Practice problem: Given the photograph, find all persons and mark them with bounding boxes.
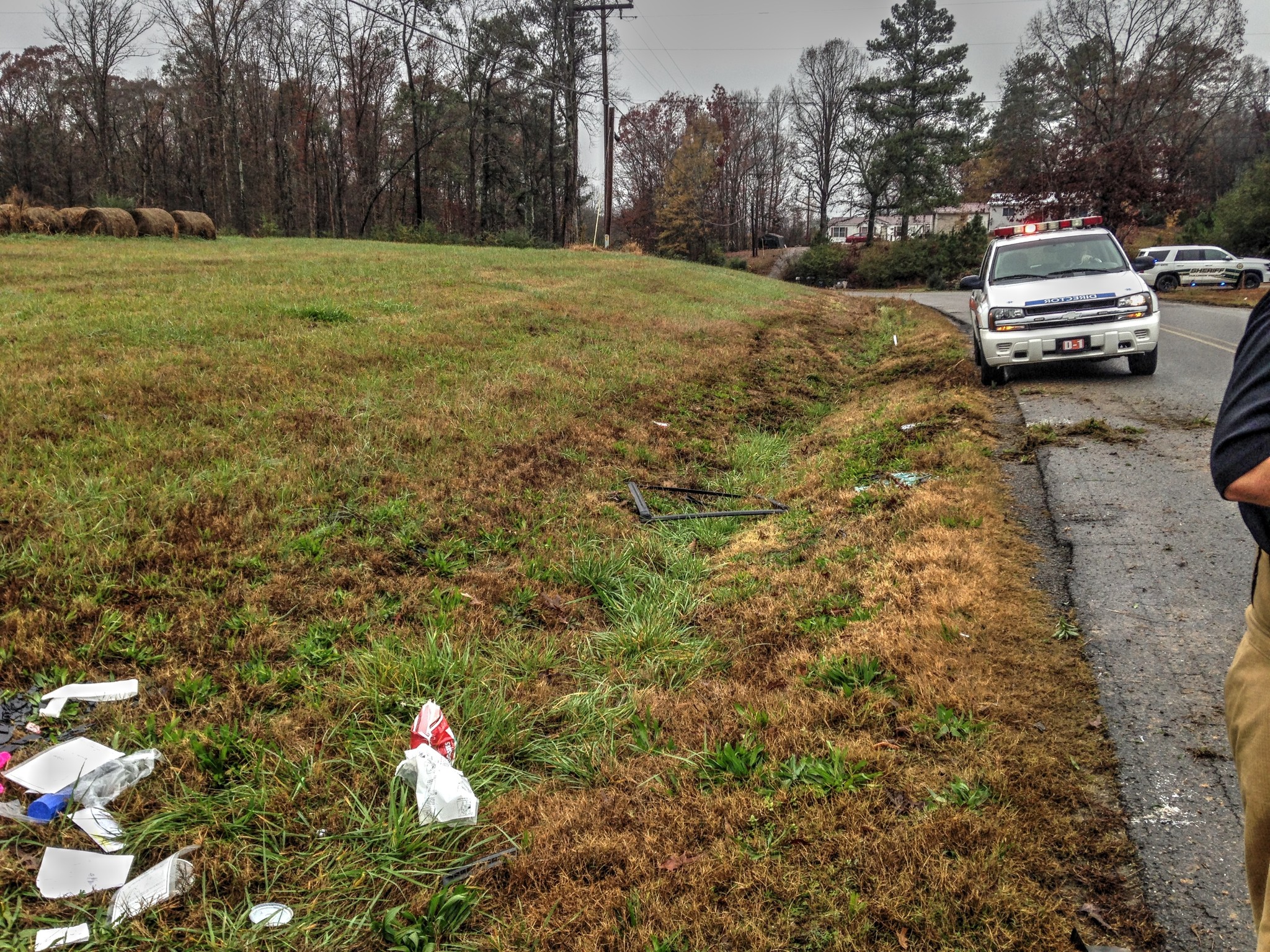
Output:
[1208,291,1270,952]
[1075,245,1102,266]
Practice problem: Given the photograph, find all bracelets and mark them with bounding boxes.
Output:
[1092,257,1095,261]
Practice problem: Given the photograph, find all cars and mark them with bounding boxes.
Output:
[958,215,1161,388]
[846,232,882,243]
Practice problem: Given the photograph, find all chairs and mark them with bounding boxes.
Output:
[998,253,1030,275]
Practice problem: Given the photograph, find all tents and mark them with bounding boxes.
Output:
[758,233,786,249]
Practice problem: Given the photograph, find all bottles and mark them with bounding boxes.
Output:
[26,793,70,824]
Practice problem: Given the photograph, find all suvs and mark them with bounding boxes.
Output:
[1137,244,1270,293]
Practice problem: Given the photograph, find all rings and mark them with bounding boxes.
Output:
[1086,257,1088,259]
